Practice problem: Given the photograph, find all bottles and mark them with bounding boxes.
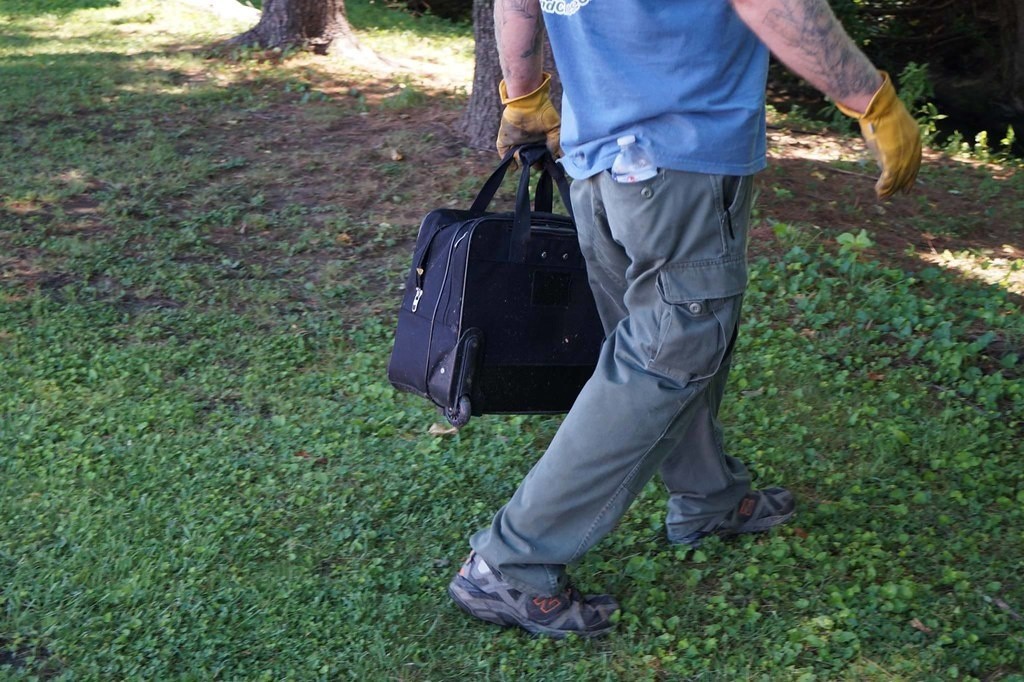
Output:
[612,134,658,183]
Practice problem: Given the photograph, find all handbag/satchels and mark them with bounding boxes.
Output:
[388,141,606,428]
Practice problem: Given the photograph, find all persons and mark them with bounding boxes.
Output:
[448,0,922,641]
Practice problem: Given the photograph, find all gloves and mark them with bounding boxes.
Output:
[833,70,921,198]
[495,69,560,161]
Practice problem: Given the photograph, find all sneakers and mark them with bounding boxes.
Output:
[448,547,620,638]
[675,486,797,543]
